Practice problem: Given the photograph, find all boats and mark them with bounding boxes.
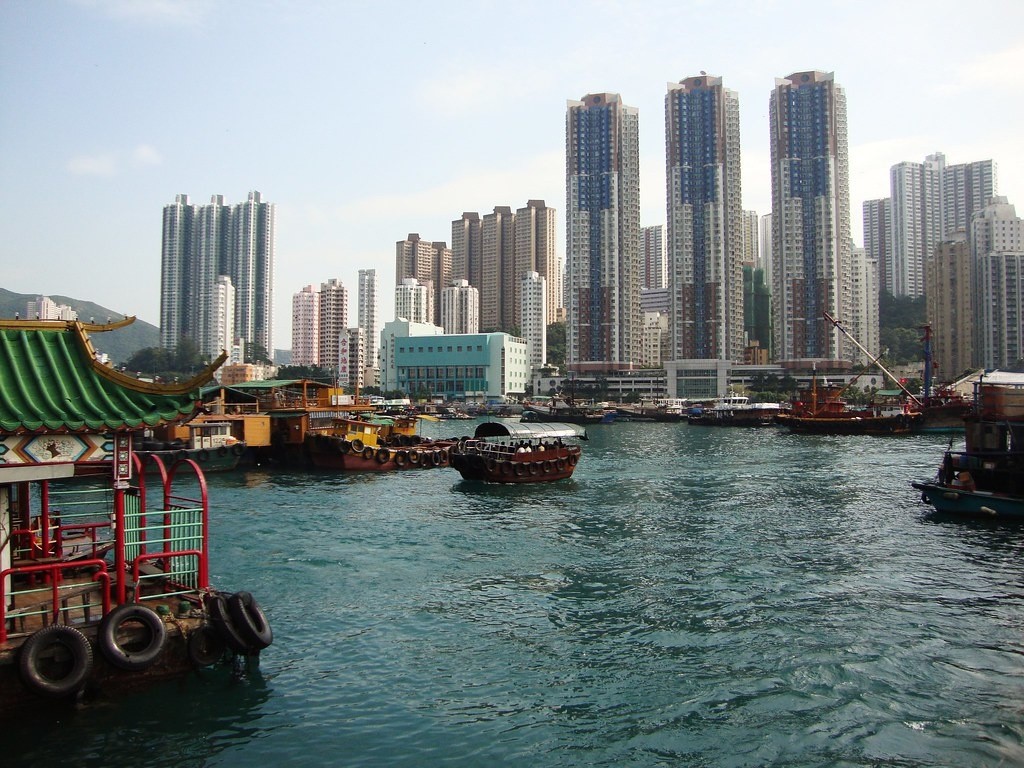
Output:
[523,398,605,423]
[911,367,1024,520]
[774,310,967,437]
[0,312,274,717]
[305,376,458,471]
[449,422,589,484]
[688,383,779,426]
[615,399,681,423]
